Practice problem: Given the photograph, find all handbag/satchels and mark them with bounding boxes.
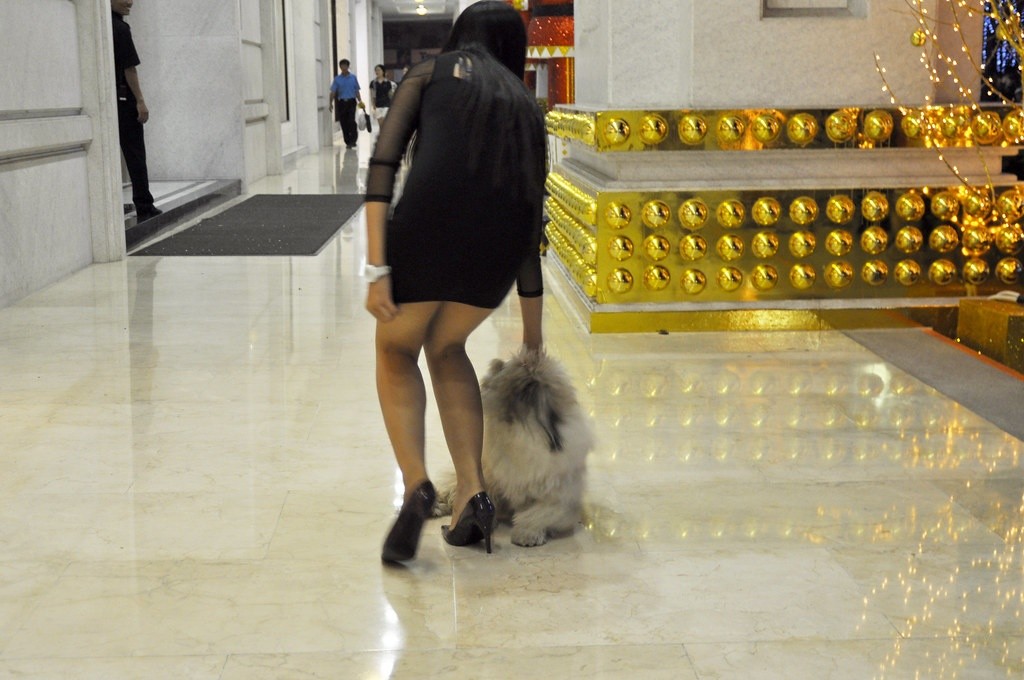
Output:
[364,109,371,132]
[359,111,367,131]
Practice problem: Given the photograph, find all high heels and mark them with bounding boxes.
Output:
[382,482,436,562]
[442,492,494,554]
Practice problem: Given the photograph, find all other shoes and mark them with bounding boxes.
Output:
[352,142,356,147]
[136,209,163,224]
[346,144,352,149]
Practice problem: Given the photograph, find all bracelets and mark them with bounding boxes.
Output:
[136,96,144,101]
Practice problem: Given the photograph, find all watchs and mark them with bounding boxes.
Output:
[364,264,392,282]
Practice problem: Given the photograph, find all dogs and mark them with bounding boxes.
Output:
[429,346,601,548]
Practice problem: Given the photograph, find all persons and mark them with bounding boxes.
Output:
[386,69,398,85]
[369,65,392,132]
[365,0,547,562]
[403,66,410,75]
[329,60,365,149]
[111,0,163,224]
